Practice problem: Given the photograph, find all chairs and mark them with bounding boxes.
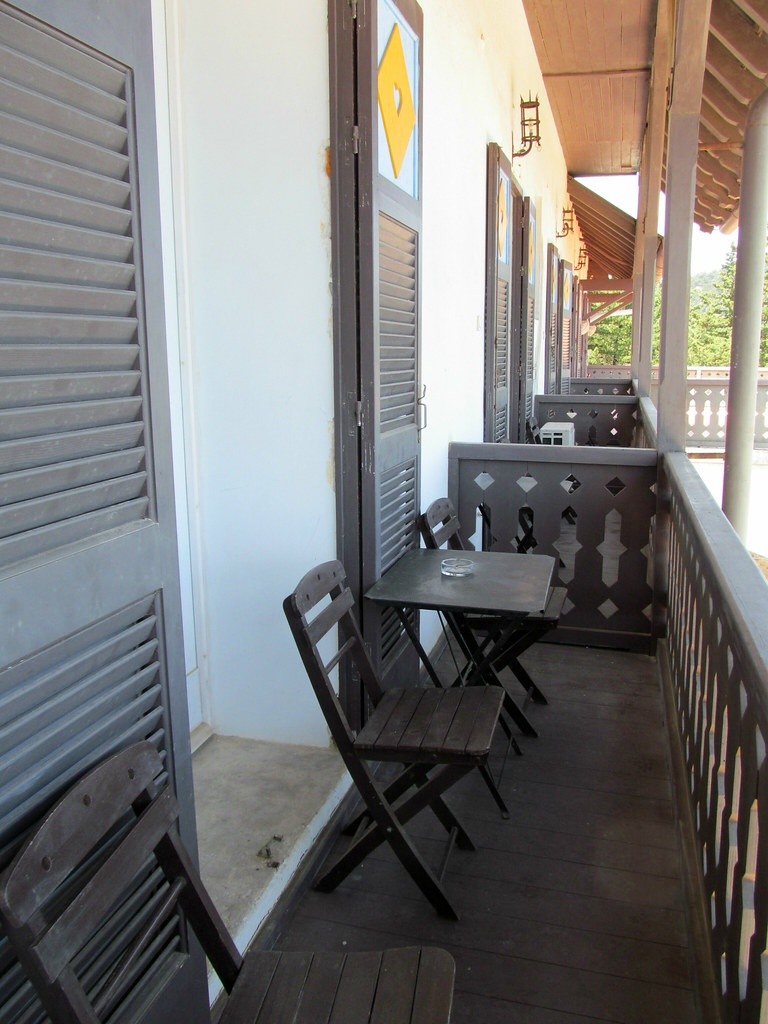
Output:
[527,416,578,526]
[416,498,569,739]
[0,740,457,1024]
[480,502,566,570]
[283,561,508,921]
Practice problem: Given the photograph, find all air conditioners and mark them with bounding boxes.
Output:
[541,420,575,447]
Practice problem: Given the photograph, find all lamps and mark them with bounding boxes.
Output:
[512,88,544,167]
[574,246,588,271]
[556,202,575,239]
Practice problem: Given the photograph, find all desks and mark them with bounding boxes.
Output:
[478,472,575,555]
[363,547,557,818]
